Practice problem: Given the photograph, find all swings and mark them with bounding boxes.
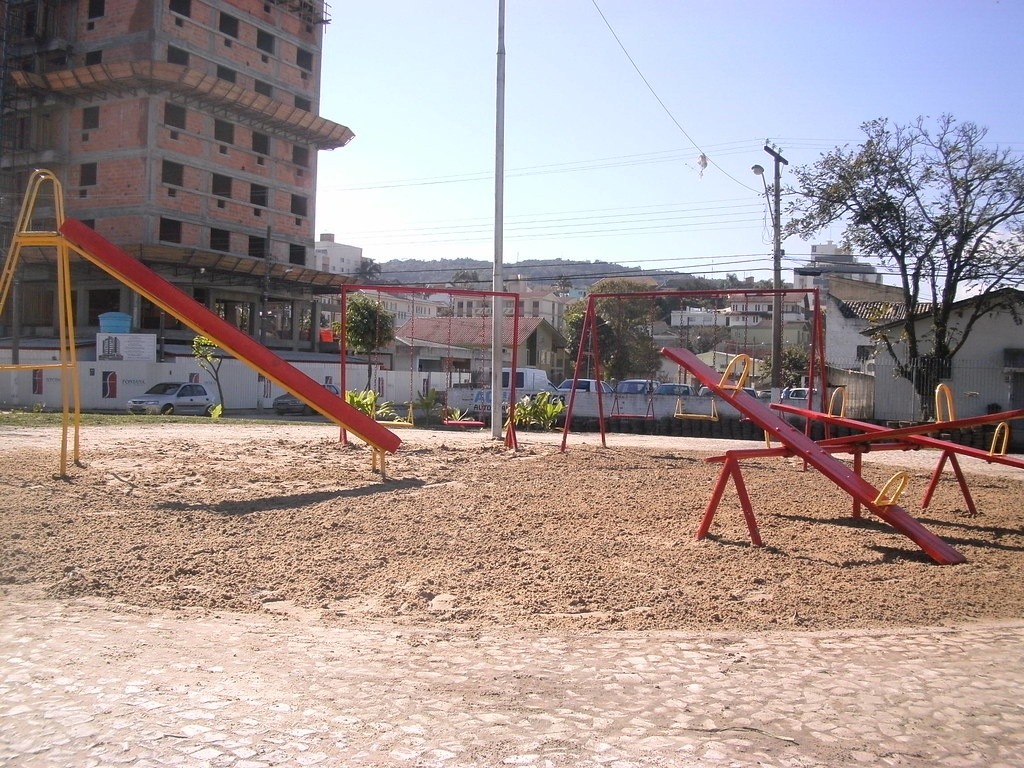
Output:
[442,293,487,427]
[673,295,719,421]
[609,293,657,420]
[739,292,786,425]
[372,288,416,429]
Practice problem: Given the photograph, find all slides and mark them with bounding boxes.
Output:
[59,215,403,456]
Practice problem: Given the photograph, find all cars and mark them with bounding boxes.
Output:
[273,383,347,417]
[698,385,758,399]
[758,390,776,398]
[557,378,614,395]
[127,381,216,417]
[612,378,662,393]
[654,383,698,397]
[789,386,817,400]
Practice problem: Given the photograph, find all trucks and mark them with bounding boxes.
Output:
[502,367,558,393]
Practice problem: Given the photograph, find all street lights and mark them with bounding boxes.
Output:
[751,136,793,407]
